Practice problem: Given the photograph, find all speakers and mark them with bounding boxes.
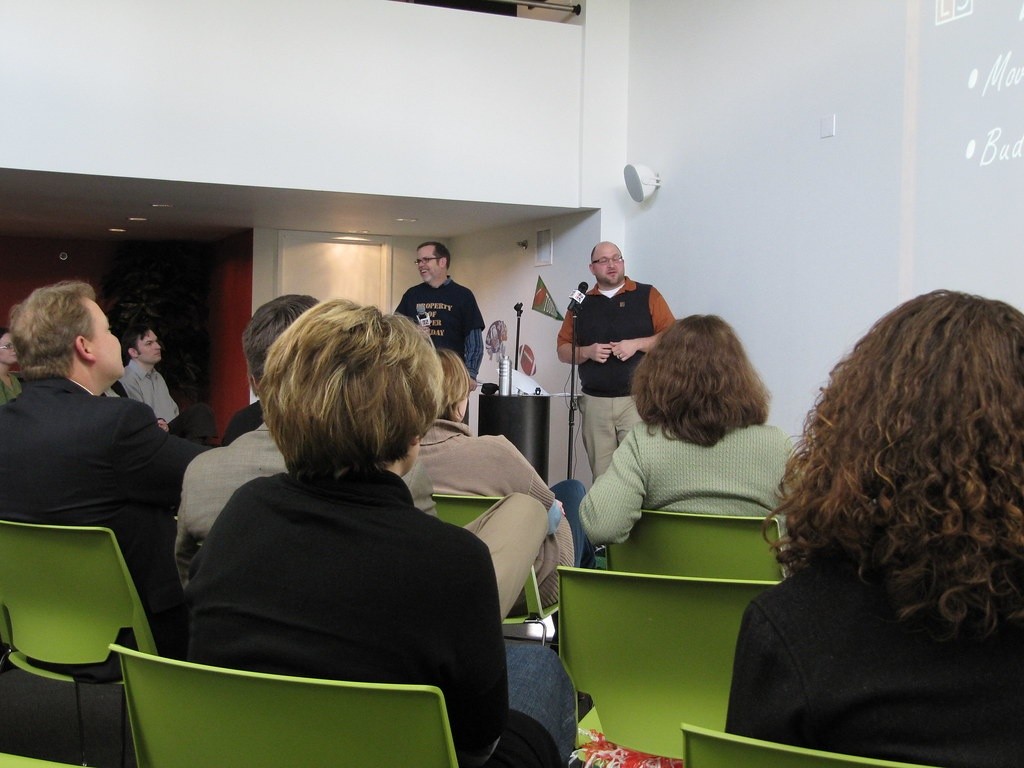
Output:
[624,164,656,202]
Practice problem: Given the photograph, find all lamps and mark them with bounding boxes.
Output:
[625,162,658,203]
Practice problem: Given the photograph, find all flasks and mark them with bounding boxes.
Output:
[498,356,512,396]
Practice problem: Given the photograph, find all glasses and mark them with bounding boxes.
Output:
[0,343,13,349]
[591,256,624,265]
[414,255,444,266]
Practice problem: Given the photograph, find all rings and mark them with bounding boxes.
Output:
[616,354,620,359]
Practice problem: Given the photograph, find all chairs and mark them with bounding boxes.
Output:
[0,513,161,768]
[680,722,943,768]
[553,565,783,763]
[430,489,558,643]
[604,498,787,586]
[106,643,462,768]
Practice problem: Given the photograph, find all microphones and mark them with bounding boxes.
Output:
[416,305,430,328]
[567,281,589,311]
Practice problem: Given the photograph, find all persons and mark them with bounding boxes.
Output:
[393,242,485,426]
[556,241,675,485]
[0,281,215,684]
[725,289,1024,768]
[417,346,587,656]
[174,294,549,624]
[579,315,796,581]
[117,323,218,446]
[0,327,23,406]
[183,297,577,768]
